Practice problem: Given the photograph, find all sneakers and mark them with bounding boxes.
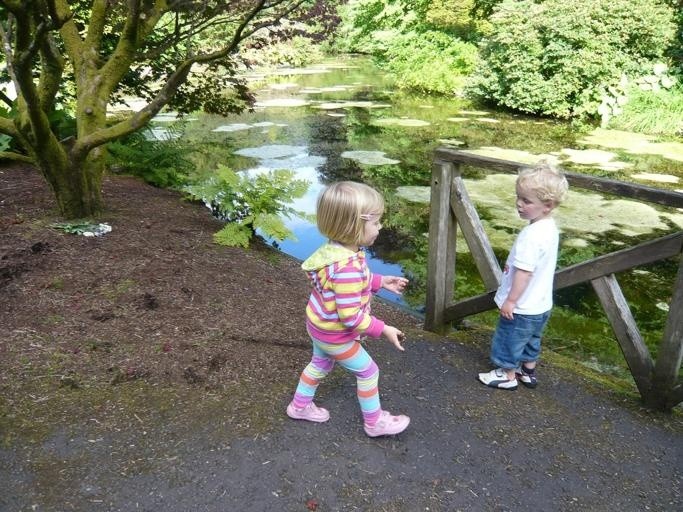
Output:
[516,365,538,389]
[476,367,519,391]
[286,400,332,423]
[362,409,411,439]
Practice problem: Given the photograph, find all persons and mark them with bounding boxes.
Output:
[477,165,569,391]
[286,181,411,438]
